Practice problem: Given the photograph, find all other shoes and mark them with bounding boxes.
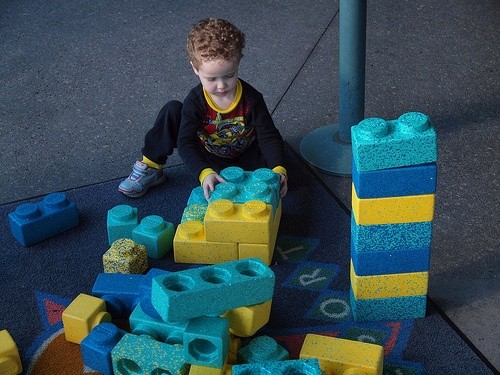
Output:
[117,160,167,197]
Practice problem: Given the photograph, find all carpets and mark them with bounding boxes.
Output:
[0,139,500,375]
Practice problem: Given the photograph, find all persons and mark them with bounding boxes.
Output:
[117,17,289,198]
